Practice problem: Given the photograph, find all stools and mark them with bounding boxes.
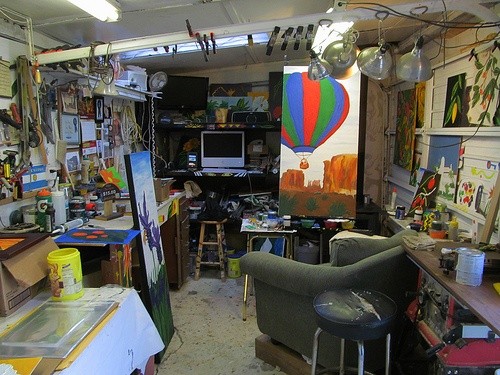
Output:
[196,219,228,281]
[312,289,397,375]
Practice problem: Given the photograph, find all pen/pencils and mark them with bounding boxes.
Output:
[88,224,102,228]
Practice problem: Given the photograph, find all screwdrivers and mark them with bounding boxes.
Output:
[3,159,10,197]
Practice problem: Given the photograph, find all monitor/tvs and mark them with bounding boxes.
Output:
[157,75,209,111]
[201,131,245,168]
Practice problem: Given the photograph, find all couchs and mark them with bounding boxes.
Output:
[241,230,418,375]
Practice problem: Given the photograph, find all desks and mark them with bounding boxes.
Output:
[0,284,166,375]
[238,207,395,265]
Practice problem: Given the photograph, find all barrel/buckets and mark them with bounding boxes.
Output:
[46,248,84,301]
[226,246,246,278]
[456,247,485,286]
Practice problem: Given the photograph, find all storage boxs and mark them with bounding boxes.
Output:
[119,71,147,91]
[154,179,176,201]
[0,237,59,318]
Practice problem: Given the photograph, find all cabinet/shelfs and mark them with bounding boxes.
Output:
[158,117,281,177]
[54,191,189,296]
[401,240,500,375]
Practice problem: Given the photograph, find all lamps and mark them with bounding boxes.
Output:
[357,43,393,80]
[68,0,123,22]
[321,40,362,80]
[307,49,334,79]
[149,71,168,91]
[397,37,434,82]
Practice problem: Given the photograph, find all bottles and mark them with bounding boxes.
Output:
[80,158,94,184]
[45,204,56,232]
[35,70,41,84]
[255,211,291,230]
[396,206,423,223]
[50,191,66,226]
[430,202,458,241]
[52,218,83,234]
[23,206,36,224]
[390,187,397,211]
[37,188,52,229]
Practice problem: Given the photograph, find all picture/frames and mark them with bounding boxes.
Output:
[94,96,105,123]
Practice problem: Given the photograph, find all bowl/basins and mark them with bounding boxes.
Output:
[342,221,354,229]
[301,220,315,229]
[324,220,337,229]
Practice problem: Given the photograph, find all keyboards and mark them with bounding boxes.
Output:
[201,168,247,173]
